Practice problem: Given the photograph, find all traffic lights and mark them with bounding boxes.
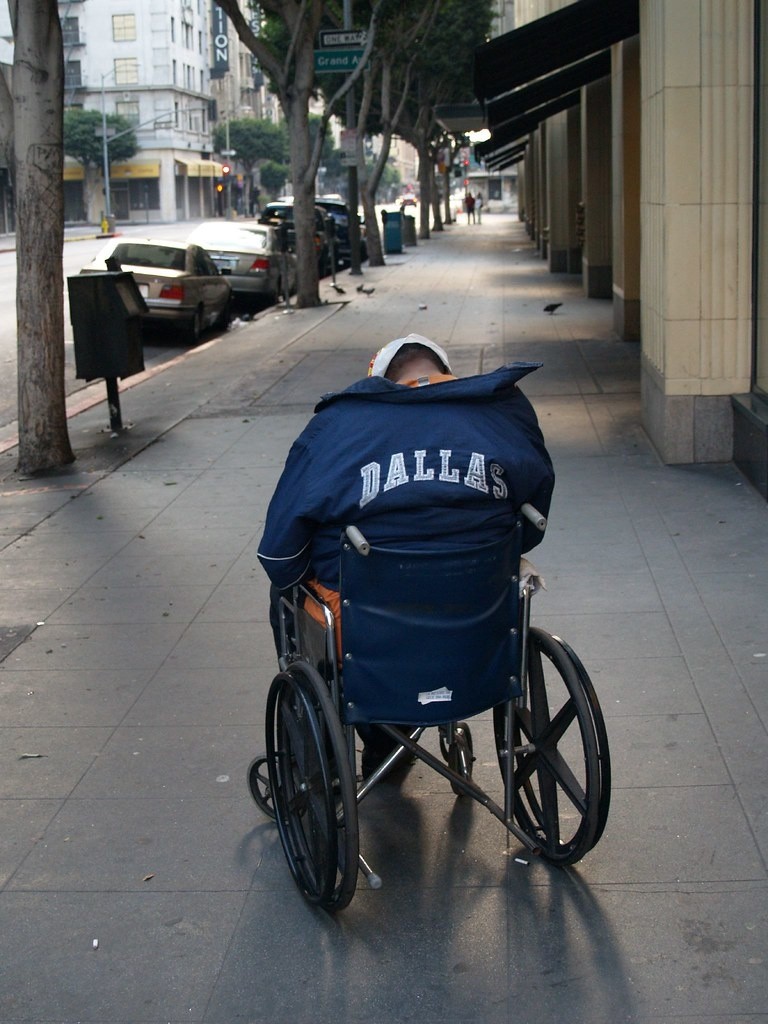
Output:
[216,181,223,195]
[222,164,232,181]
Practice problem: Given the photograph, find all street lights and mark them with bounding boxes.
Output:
[101,61,140,233]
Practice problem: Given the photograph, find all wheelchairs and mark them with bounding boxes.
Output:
[248,502,614,916]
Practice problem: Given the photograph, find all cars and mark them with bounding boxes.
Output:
[402,194,418,208]
[188,220,300,309]
[79,236,233,342]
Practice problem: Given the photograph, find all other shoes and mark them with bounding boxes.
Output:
[359,747,410,788]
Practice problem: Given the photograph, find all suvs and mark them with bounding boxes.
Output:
[315,196,368,265]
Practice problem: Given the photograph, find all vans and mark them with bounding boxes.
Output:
[256,202,344,282]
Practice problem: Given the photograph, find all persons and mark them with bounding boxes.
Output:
[465,192,476,225]
[475,193,483,224]
[256,335,557,787]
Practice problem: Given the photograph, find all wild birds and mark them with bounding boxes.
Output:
[330,283,345,294]
[543,303,562,314]
[357,284,375,297]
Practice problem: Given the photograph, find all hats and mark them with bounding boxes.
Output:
[368,332,454,383]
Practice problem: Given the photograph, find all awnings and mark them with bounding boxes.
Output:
[459,1,641,174]
